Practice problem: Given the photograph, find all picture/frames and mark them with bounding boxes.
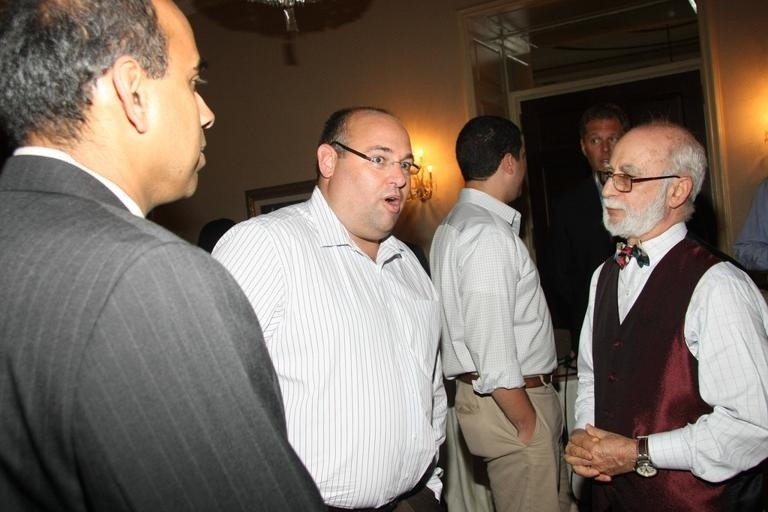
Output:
[244,178,317,219]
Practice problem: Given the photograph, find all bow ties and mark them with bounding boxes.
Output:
[613,242,649,270]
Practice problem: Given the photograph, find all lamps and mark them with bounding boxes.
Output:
[406,146,434,203]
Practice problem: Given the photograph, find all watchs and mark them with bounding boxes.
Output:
[632,435,659,479]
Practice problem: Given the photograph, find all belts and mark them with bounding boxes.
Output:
[457,373,552,388]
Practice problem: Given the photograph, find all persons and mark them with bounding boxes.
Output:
[429,115,579,512]
[540,101,717,353]
[0,0,327,512]
[563,120,768,512]
[211,106,449,512]
[730,176,768,274]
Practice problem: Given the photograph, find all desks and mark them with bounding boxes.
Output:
[434,359,597,512]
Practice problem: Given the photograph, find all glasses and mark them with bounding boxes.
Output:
[597,171,680,192]
[329,141,420,175]
